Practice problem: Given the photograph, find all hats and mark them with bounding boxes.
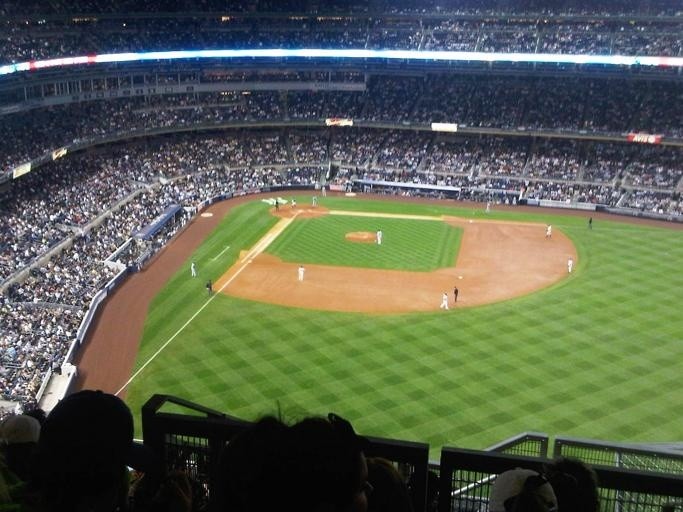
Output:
[488,467,558,511]
[0,414,41,444]
